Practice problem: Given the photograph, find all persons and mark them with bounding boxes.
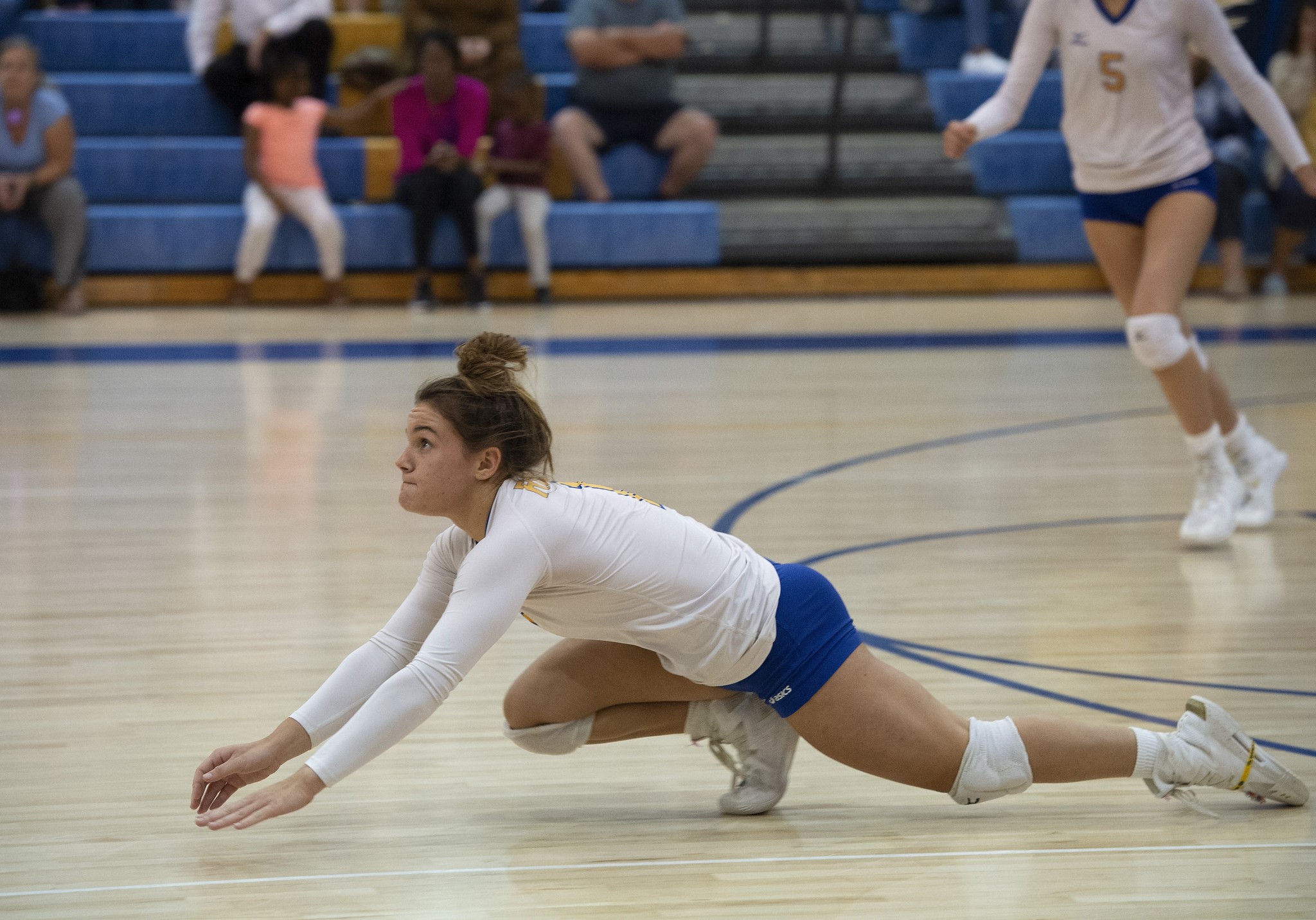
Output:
[942,0,1316,549]
[181,0,717,316]
[0,35,89,314]
[189,330,1310,832]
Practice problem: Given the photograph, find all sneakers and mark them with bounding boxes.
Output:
[1144,694,1311,819]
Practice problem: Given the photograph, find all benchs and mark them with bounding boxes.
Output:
[0,0,1298,270]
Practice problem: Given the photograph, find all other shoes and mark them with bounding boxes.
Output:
[1178,462,1244,546]
[1233,438,1289,528]
[410,280,433,308]
[528,286,550,304]
[461,274,484,313]
[212,281,255,307]
[706,691,800,815]
[325,280,351,310]
